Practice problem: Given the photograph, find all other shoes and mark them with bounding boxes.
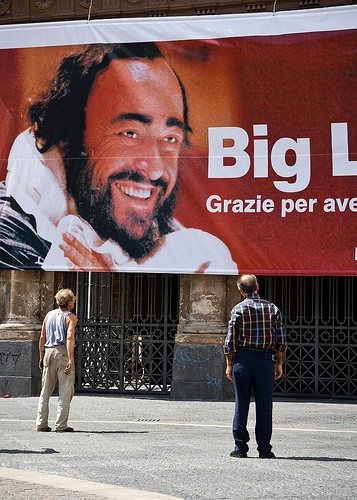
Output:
[39,427,52,432]
[259,452,275,458]
[55,427,74,432]
[229,450,247,458]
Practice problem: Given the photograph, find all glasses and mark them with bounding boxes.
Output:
[72,296,77,301]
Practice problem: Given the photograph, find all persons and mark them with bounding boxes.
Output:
[223,274,287,458]
[0,42,239,274]
[36,289,77,432]
